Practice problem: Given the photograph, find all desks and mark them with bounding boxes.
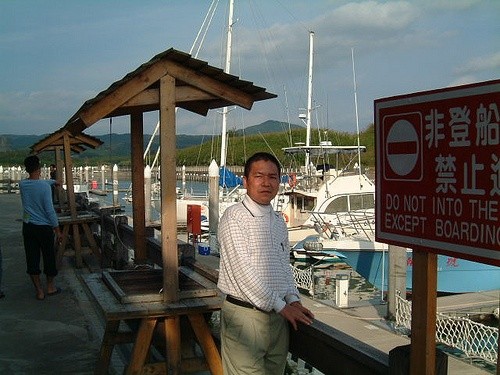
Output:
[81,265,224,375]
[53,202,103,267]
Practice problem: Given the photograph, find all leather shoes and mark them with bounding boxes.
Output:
[226,296,262,310]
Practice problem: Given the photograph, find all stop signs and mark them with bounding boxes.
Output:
[386,119,420,176]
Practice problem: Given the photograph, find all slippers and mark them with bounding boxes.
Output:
[48,287,61,296]
[36,295,46,301]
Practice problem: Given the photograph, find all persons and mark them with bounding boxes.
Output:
[217,152,314,375]
[19,155,64,300]
[50,164,56,180]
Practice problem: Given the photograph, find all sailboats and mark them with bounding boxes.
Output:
[122,0,499,293]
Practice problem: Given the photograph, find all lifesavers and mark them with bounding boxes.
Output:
[280,212,289,224]
[287,174,297,188]
